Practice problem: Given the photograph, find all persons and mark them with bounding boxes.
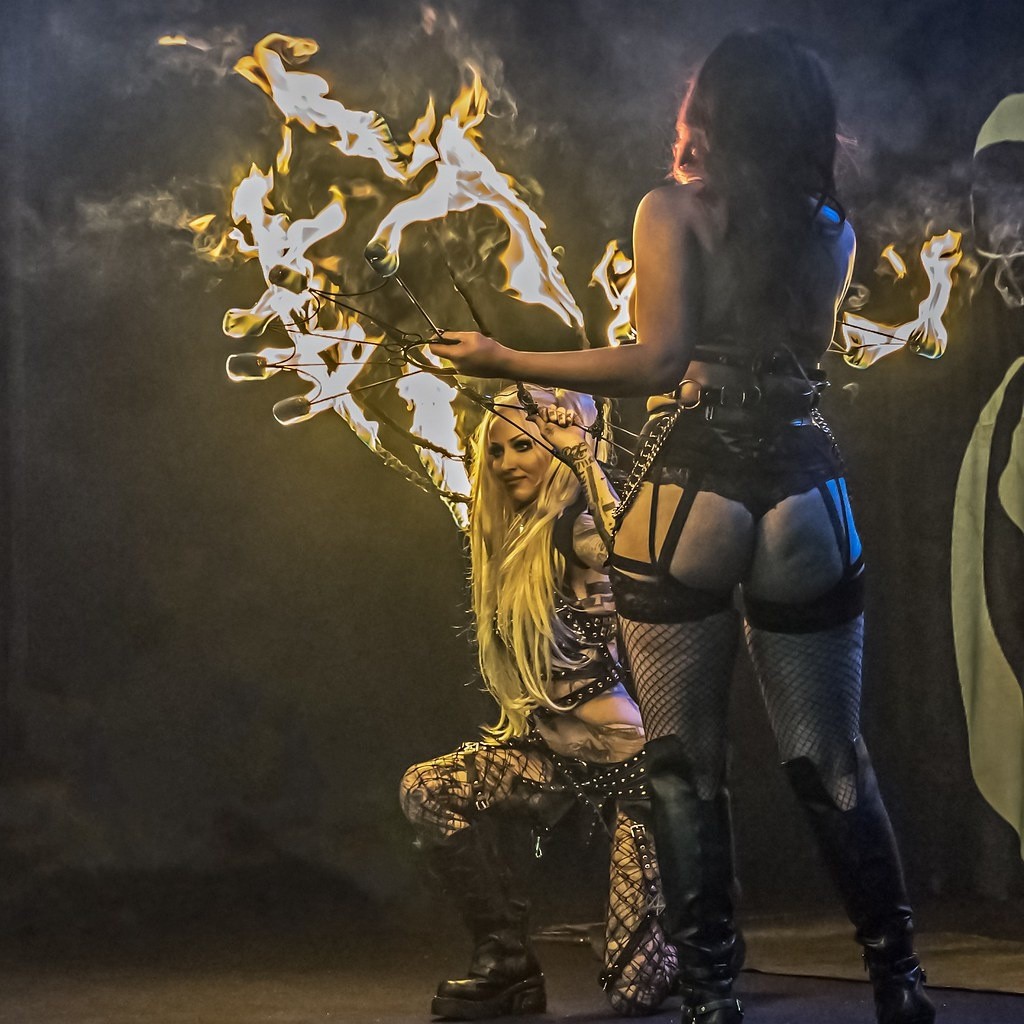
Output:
[400,383,684,1021]
[432,30,943,1024]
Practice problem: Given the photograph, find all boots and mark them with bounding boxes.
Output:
[780,732,937,1024]
[643,732,746,1024]
[431,827,547,1018]
